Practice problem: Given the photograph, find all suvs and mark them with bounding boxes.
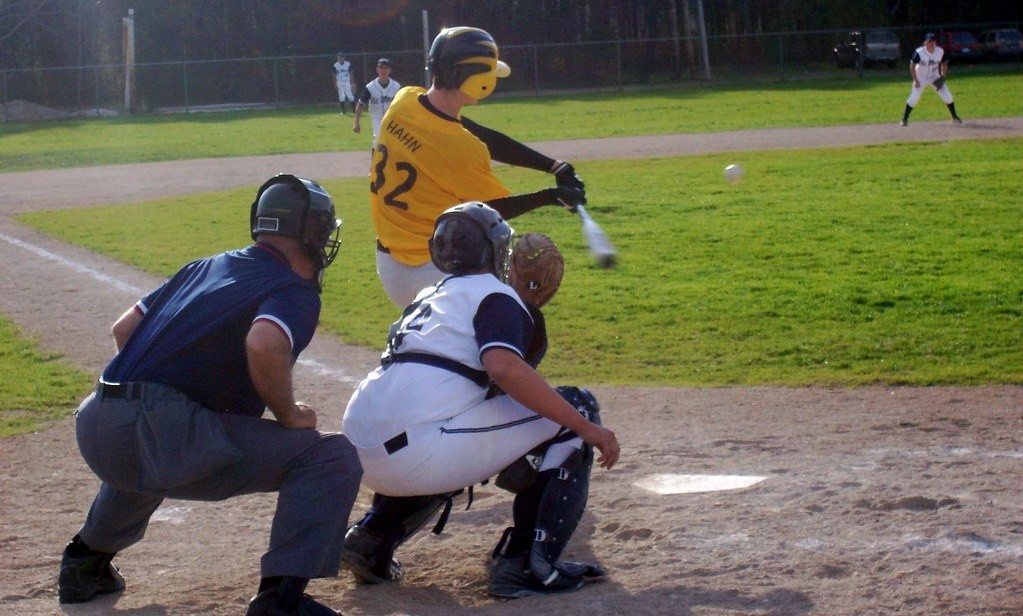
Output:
[833,30,902,70]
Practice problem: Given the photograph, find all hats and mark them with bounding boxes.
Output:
[376,59,392,69]
[926,33,937,41]
[337,52,345,57]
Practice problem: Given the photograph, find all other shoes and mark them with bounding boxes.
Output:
[952,117,963,124]
[901,118,908,125]
[341,111,345,116]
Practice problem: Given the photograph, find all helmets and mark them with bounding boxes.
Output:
[428,200,516,274]
[248,175,343,238]
[428,26,512,99]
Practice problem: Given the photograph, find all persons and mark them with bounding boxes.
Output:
[340,201,621,601]
[332,53,356,115]
[353,58,402,177]
[59,171,364,616]
[370,26,586,309]
[900,34,962,126]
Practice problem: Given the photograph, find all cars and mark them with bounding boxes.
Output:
[984,29,1023,61]
[937,31,980,64]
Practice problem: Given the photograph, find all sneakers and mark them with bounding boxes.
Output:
[339,525,403,583]
[488,556,584,599]
[244,589,341,616]
[59,543,127,604]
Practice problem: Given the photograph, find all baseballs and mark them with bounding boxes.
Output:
[724,164,744,185]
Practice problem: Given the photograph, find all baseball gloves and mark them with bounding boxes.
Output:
[507,234,565,310]
[932,75,945,91]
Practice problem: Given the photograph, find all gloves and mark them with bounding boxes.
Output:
[557,186,588,213]
[552,163,585,190]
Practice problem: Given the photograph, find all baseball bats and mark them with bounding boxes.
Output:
[574,201,620,271]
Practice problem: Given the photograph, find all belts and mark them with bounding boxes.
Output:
[385,431,407,455]
[375,238,391,253]
[97,375,140,400]
